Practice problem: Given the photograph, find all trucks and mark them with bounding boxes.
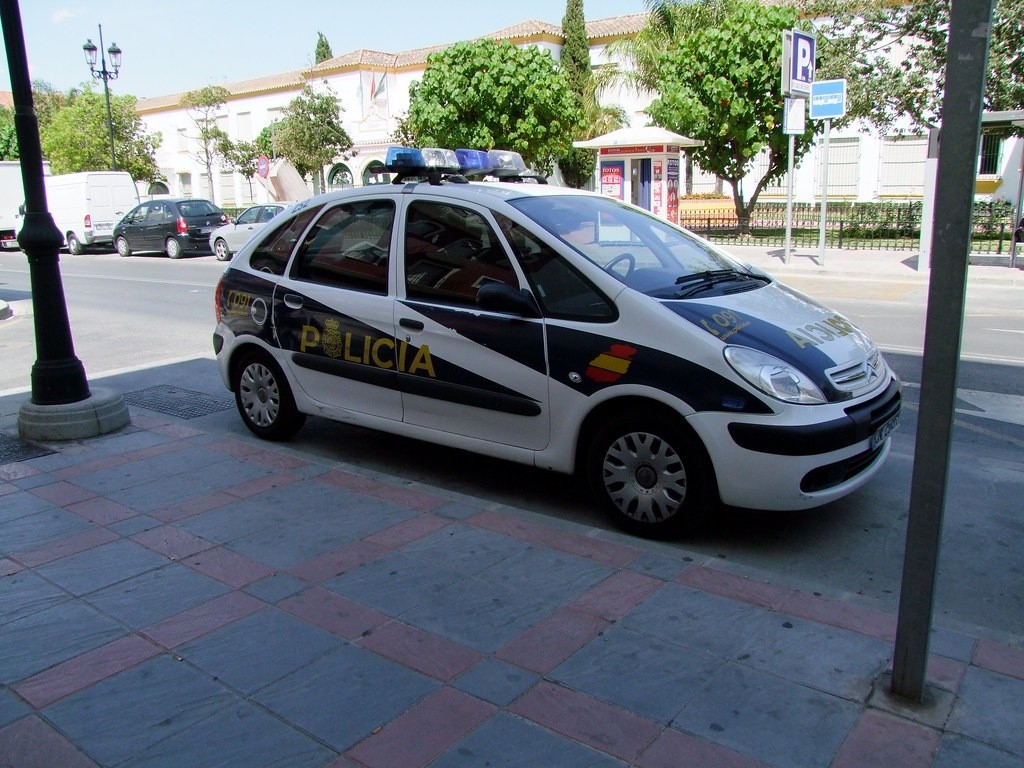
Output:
[0,160,52,251]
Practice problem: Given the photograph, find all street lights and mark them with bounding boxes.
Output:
[83,22,122,169]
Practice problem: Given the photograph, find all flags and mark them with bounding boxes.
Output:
[371,75,376,100]
[373,73,388,100]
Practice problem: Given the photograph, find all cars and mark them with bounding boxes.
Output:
[209,200,297,262]
[212,144,901,542]
[113,196,231,260]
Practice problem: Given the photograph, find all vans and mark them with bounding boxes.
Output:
[16,171,143,256]
